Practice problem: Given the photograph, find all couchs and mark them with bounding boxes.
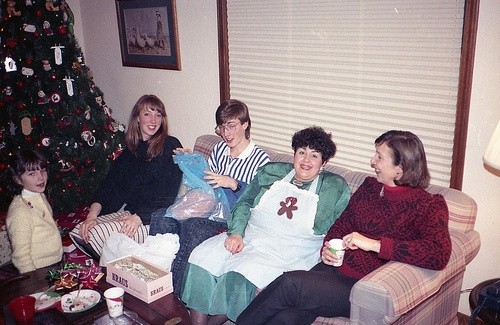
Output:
[166,135,480,325]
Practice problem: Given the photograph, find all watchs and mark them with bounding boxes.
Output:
[233,183,241,192]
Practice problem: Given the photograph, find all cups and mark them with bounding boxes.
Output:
[104,287,124,318]
[8,296,36,323]
[328,238,347,266]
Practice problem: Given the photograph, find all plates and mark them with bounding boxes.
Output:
[56,289,101,313]
[28,292,60,312]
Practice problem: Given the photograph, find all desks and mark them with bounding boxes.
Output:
[0,261,191,325]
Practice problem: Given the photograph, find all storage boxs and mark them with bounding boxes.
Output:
[106,255,173,304]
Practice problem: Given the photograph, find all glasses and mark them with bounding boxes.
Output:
[214,123,242,132]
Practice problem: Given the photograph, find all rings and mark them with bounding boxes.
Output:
[347,238,351,242]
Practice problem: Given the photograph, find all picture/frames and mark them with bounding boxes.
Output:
[115,0,181,71]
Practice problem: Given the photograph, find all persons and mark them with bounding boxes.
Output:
[69,94,185,267]
[178,125,351,325]
[149,99,270,301]
[236,129,452,325]
[5,149,64,275]
